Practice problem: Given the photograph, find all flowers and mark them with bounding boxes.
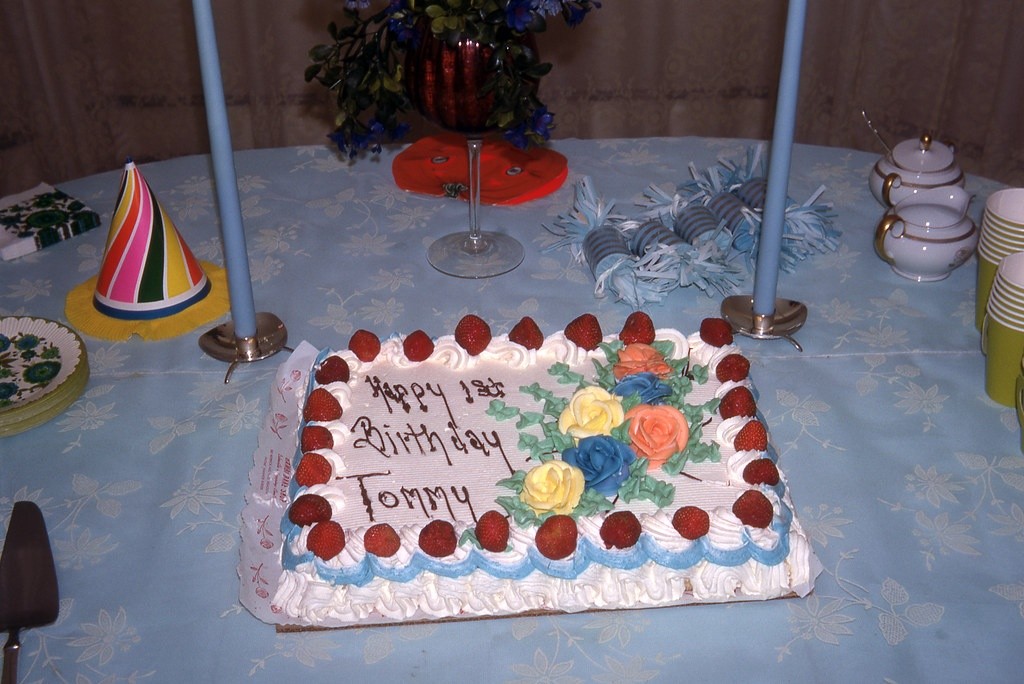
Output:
[304,0,601,164]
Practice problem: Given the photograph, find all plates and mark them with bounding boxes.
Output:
[0,315,91,438]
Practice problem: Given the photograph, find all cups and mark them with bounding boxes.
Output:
[985,252,1024,409]
[975,187,1024,335]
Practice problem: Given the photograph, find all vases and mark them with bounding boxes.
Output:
[404,23,528,278]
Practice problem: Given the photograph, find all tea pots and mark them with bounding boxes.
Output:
[868,134,965,209]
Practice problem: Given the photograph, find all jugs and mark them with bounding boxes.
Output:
[874,185,980,282]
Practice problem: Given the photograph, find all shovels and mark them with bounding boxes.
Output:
[0,497,62,684]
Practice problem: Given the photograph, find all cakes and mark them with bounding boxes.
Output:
[271,310,810,622]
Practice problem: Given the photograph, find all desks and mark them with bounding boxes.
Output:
[0,134,1024,684]
